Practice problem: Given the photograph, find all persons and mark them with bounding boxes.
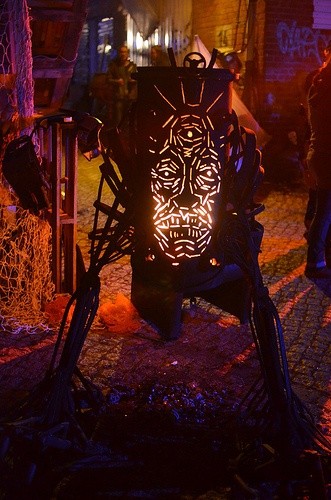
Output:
[150,45,173,68]
[105,44,140,129]
[303,31,331,307]
